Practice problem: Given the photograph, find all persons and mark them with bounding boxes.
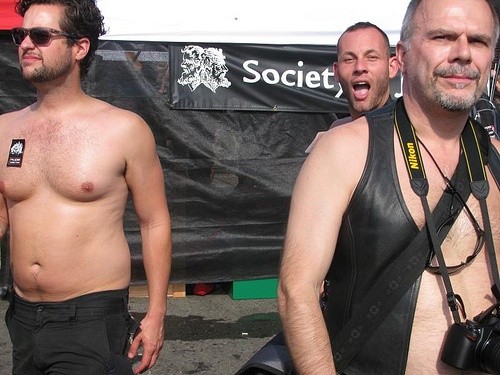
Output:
[277,0,500,375]
[0,0,172,375]
[329,21,399,130]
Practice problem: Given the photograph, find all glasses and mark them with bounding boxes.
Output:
[9,26,81,47]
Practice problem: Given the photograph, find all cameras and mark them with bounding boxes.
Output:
[440,311,500,375]
